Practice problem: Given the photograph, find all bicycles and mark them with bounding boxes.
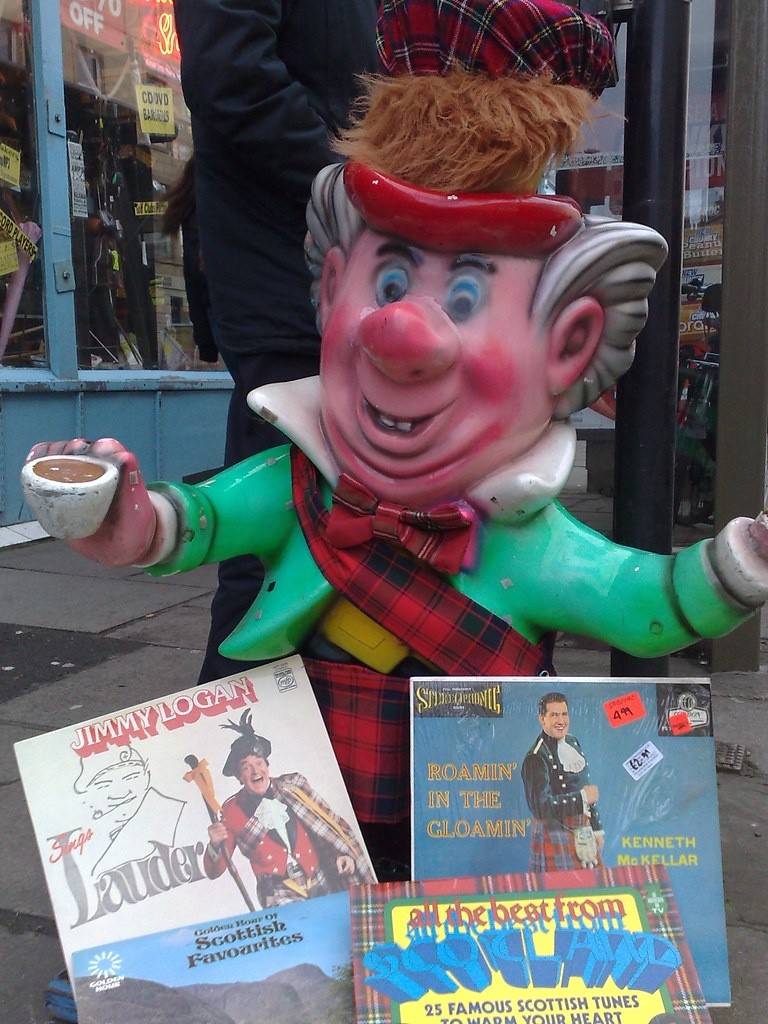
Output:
[671,283,721,525]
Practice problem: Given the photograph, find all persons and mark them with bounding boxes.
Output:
[521,692,605,873]
[202,734,375,908]
[174,0,372,682]
[12,656,378,1024]
[162,153,219,363]
[25,88,767,1022]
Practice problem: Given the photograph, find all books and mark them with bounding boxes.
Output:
[349,866,712,1024]
[408,676,732,1007]
